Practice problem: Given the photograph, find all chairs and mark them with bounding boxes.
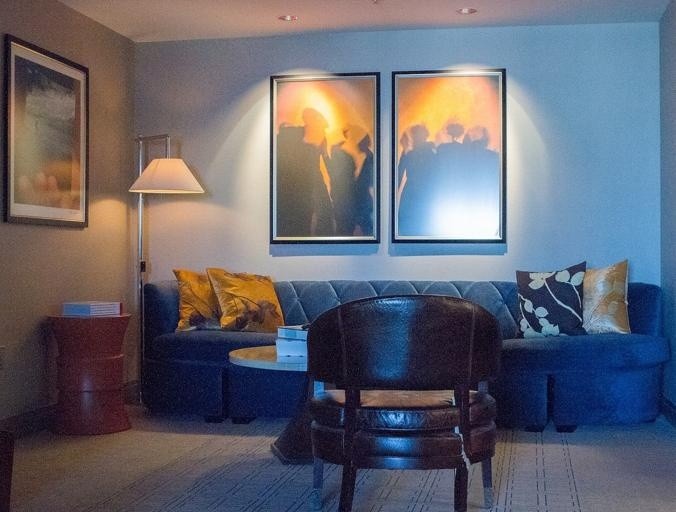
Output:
[306,295,502,512]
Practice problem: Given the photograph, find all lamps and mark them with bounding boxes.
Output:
[125,133,204,408]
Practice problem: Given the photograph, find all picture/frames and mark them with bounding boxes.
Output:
[3,34,89,228]
[270,72,380,244]
[392,69,506,243]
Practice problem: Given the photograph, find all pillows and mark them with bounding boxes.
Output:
[206,267,285,333]
[173,268,223,333]
[582,259,632,334]
[512,261,588,338]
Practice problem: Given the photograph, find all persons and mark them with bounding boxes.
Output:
[18,171,68,209]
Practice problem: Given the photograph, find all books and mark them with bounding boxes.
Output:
[273,338,307,358]
[60,301,121,318]
[275,323,311,341]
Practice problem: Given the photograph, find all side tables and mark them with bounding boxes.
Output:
[46,312,131,435]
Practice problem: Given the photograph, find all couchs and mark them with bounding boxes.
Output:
[139,279,671,433]
